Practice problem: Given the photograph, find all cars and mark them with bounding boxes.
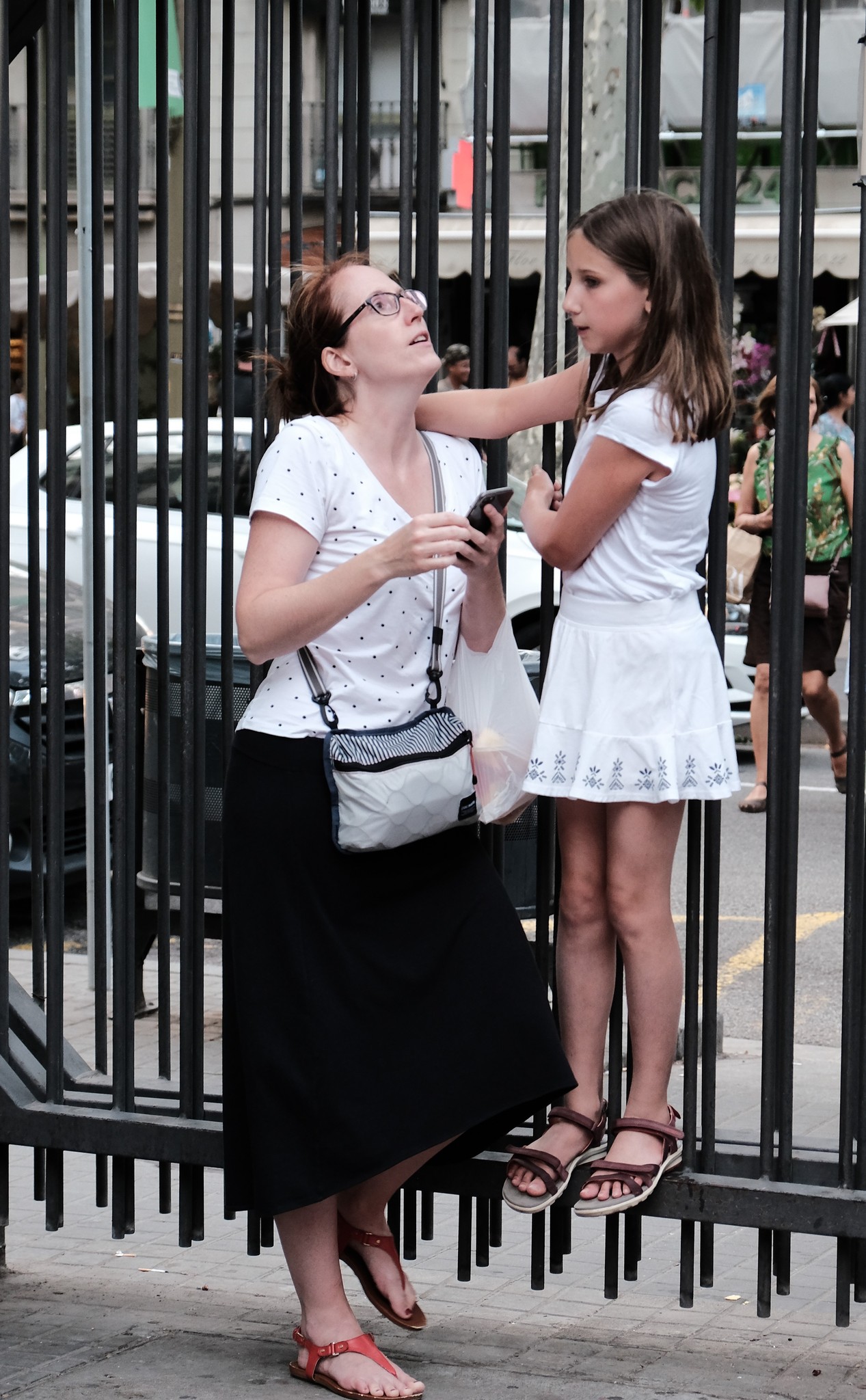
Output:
[6,558,149,874]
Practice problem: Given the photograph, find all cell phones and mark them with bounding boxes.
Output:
[456,486,514,560]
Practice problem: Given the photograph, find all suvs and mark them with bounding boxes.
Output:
[8,415,760,757]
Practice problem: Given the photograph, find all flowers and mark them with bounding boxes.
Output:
[730,300,780,406]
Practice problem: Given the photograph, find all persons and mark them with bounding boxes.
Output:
[235,330,254,418]
[815,374,854,454]
[734,374,854,814]
[228,255,576,1400]
[417,194,744,1219]
[437,343,527,392]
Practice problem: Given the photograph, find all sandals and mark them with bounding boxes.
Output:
[287,1324,424,1400]
[334,1209,428,1330]
[828,729,847,794]
[502,1107,610,1214]
[738,781,768,813]
[574,1102,684,1217]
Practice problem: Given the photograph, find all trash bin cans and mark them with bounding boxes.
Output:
[136,633,269,903]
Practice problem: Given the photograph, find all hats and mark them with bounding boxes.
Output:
[236,328,266,363]
[440,343,471,366]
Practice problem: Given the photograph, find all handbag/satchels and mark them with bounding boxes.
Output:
[768,575,829,617]
[706,522,762,604]
[326,704,479,853]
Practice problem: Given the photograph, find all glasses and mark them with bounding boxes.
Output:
[336,289,429,338]
